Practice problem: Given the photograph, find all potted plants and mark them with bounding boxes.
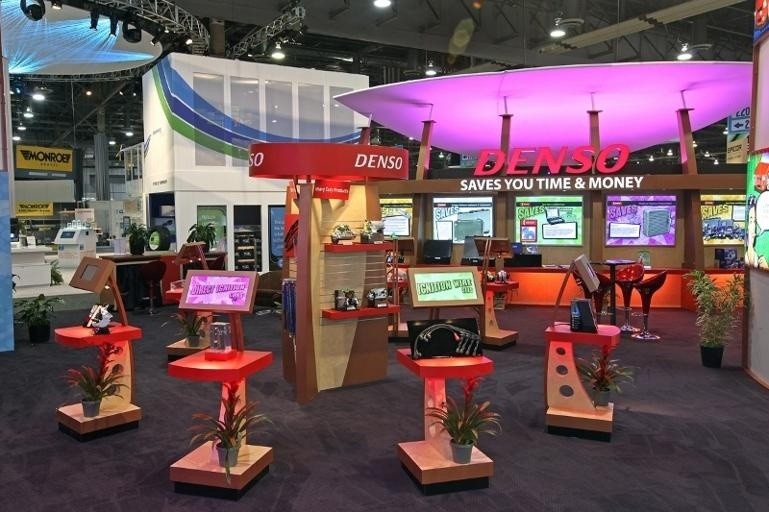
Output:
[62,343,134,416]
[14,291,66,344]
[186,223,216,254]
[680,268,748,370]
[576,345,634,410]
[160,309,214,348]
[186,378,279,490]
[423,377,502,464]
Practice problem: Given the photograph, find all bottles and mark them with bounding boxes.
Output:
[72,220,86,229]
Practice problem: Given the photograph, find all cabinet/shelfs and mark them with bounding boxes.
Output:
[322,241,401,322]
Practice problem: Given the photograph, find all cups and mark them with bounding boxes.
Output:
[67,223,71,229]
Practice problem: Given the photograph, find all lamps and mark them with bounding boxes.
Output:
[547,10,567,40]
[676,42,694,62]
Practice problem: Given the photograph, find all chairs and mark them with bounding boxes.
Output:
[595,270,610,327]
[631,267,668,340]
[615,260,647,335]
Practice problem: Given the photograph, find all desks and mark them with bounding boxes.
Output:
[591,257,638,325]
